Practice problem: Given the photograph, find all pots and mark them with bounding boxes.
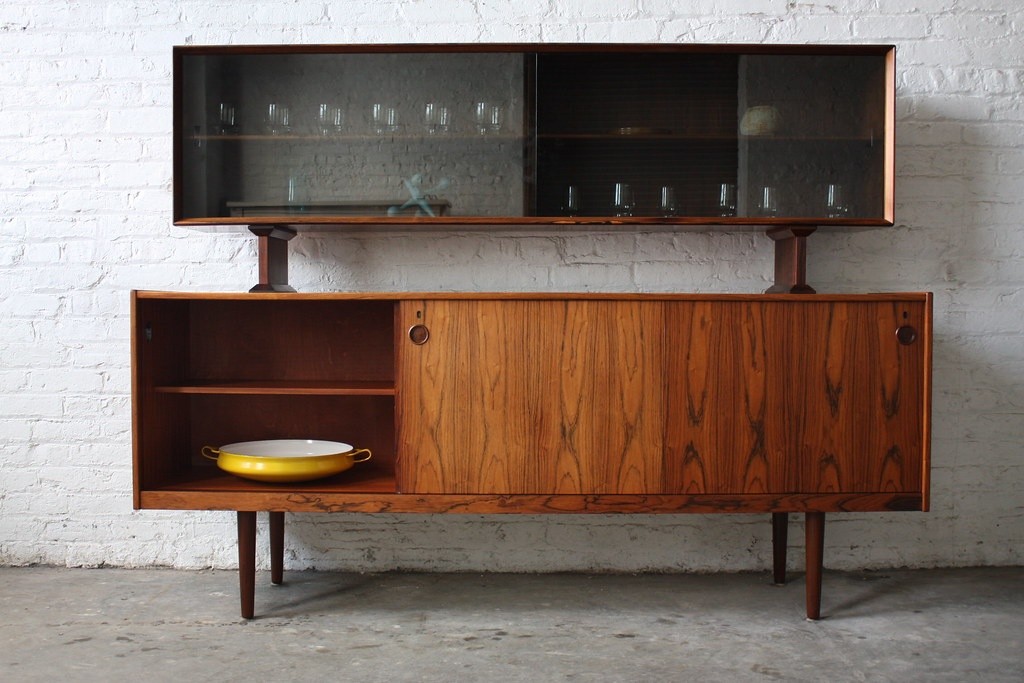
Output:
[201,439,372,483]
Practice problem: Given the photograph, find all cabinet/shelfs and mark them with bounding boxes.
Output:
[132,45,933,620]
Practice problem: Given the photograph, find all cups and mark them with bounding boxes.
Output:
[720,182,739,218]
[315,102,347,136]
[423,101,453,135]
[214,101,240,136]
[740,105,786,137]
[613,183,637,216]
[473,101,504,135]
[263,103,292,136]
[763,187,783,218]
[661,185,681,217]
[364,103,399,136]
[560,184,581,216]
[826,184,851,218]
